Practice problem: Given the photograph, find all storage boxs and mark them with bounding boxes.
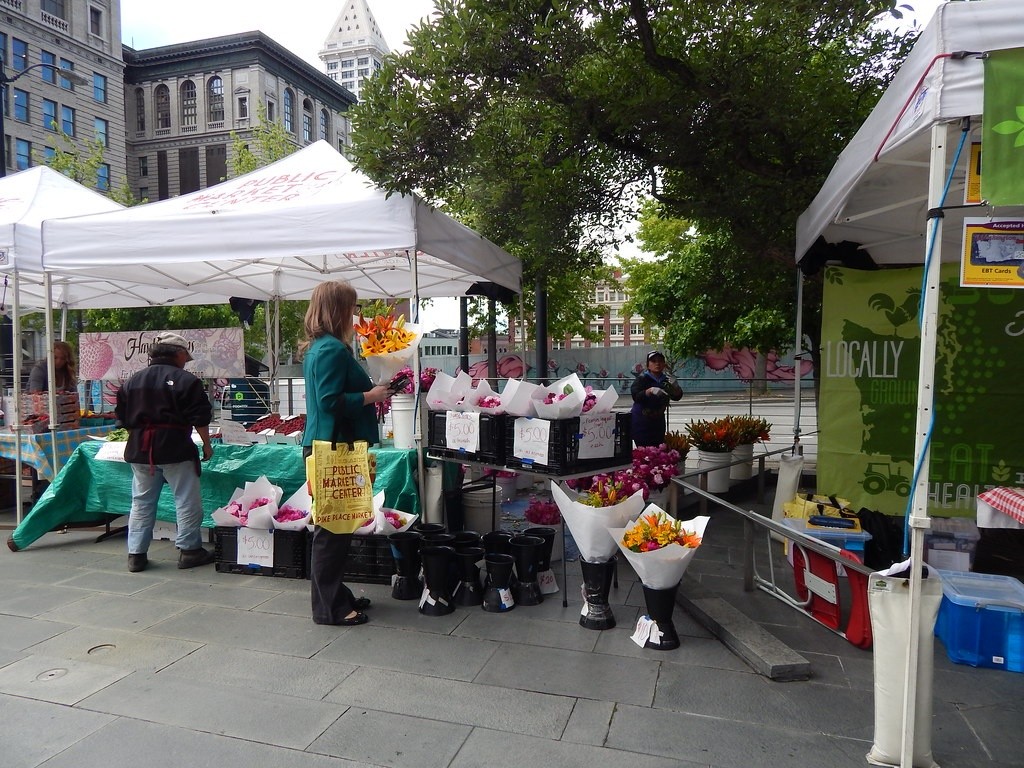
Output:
[506,412,633,477]
[937,569,1024,673]
[923,517,981,572]
[80,418,116,427]
[245,412,307,445]
[19,391,80,435]
[781,517,873,568]
[427,409,509,466]
[213,525,396,586]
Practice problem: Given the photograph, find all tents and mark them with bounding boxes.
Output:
[794,0,1024,768]
[40,142,525,522]
[0,164,274,527]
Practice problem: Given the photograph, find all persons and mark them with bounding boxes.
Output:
[24,343,77,393]
[303,280,395,625]
[631,351,684,449]
[115,337,215,570]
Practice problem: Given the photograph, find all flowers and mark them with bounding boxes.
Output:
[352,300,414,359]
[222,496,408,529]
[374,365,773,554]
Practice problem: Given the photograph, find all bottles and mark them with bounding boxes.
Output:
[463,484,502,535]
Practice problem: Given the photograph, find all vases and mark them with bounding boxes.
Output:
[388,521,557,616]
[579,554,619,630]
[640,580,681,651]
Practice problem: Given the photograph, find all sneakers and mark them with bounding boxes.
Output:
[126,554,148,572]
[177,546,215,569]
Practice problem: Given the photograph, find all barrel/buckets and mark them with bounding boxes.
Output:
[516,473,534,489]
[485,475,516,502]
[528,522,563,560]
[391,393,428,449]
[729,444,754,480]
[696,450,733,493]
[424,468,444,524]
[672,461,685,494]
[641,485,671,517]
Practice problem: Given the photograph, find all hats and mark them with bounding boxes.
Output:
[647,351,666,370]
[156,332,195,363]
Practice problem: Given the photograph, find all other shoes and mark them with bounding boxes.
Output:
[333,608,368,625]
[356,596,371,612]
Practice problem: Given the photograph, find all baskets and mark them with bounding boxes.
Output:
[10,414,48,435]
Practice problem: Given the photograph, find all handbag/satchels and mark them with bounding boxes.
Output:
[306,396,377,534]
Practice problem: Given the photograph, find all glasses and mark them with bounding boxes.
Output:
[354,303,362,312]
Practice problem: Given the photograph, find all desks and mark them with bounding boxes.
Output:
[6,439,420,552]
[977,488,1024,529]
[0,425,117,512]
